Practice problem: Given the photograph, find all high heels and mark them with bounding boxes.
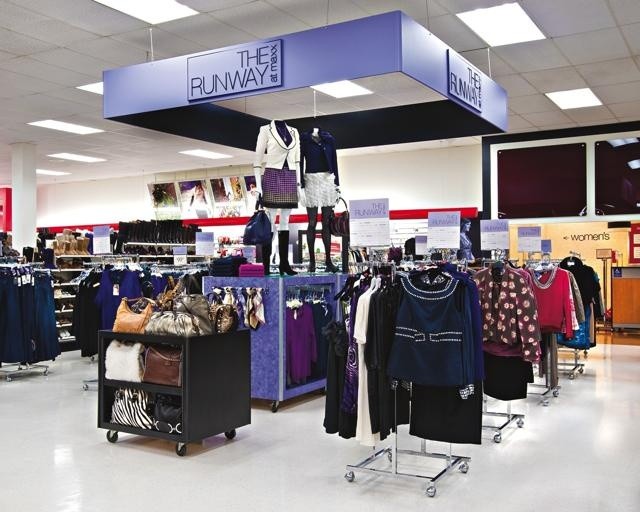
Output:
[158,247,164,255]
[58,328,76,340]
[148,246,157,255]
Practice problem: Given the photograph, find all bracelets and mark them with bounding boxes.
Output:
[297,183,301,186]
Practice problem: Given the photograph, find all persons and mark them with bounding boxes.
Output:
[253,118,301,276]
[460,218,475,260]
[300,127,340,273]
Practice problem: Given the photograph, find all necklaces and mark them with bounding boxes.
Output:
[277,127,287,142]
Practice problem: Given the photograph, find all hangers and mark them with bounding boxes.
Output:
[492,249,518,271]
[562,253,584,271]
[70,257,103,288]
[409,249,450,279]
[104,255,132,272]
[334,262,389,301]
[287,286,328,301]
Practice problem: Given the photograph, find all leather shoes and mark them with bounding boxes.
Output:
[127,246,147,255]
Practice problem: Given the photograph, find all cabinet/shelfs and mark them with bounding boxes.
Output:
[203,277,343,411]
[51,255,103,342]
[98,328,251,456]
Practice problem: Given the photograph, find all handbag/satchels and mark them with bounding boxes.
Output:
[141,343,183,388]
[112,297,159,335]
[243,210,271,245]
[151,393,182,435]
[145,299,205,337]
[206,292,240,334]
[109,386,157,430]
[159,274,217,336]
[104,338,145,384]
[329,211,349,237]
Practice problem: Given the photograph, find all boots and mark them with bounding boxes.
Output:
[71,235,78,255]
[342,236,349,274]
[53,241,59,255]
[77,237,87,255]
[262,232,273,275]
[307,224,316,272]
[23,246,33,262]
[58,242,64,255]
[41,249,57,269]
[278,230,298,277]
[65,241,75,255]
[321,230,339,273]
[125,220,201,244]
[82,237,90,255]
[116,222,127,254]
